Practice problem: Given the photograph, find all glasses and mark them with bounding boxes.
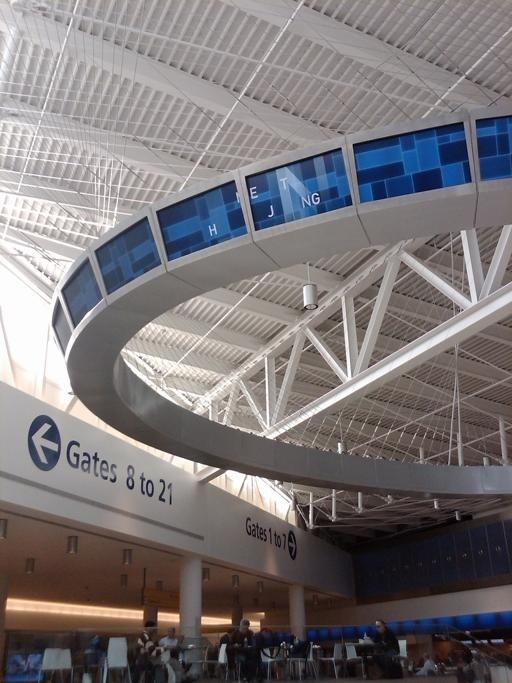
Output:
[375,625,380,628]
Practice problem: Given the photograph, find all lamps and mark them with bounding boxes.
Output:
[122,549,132,565]
[257,582,263,593]
[66,535,75,553]
[24,558,34,574]
[202,567,209,581]
[156,581,163,591]
[231,575,239,588]
[121,575,127,584]
[303,261,318,309]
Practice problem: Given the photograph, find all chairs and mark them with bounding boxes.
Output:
[6,639,237,682]
[237,639,409,682]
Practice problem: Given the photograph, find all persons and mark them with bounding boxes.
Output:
[371,618,402,679]
[138,621,179,660]
[221,618,257,683]
